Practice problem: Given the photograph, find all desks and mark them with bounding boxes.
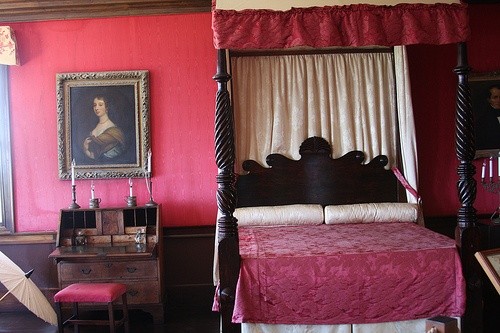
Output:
[476,218,500,250]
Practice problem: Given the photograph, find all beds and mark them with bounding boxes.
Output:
[212,0,477,333]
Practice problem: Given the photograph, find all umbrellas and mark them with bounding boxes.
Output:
[0,250,58,327]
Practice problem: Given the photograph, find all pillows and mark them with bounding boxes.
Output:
[324,202,418,225]
[233,204,325,227]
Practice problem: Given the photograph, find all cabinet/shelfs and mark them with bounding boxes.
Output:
[47,203,166,333]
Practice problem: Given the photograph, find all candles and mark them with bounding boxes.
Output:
[130,182,133,197]
[481,162,486,179]
[497,152,500,177]
[489,156,493,178]
[91,187,96,200]
[147,148,152,172]
[71,158,76,185]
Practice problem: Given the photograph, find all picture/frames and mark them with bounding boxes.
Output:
[56,68,152,180]
[469,72,500,161]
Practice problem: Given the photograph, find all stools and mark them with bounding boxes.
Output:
[54,282,130,333]
